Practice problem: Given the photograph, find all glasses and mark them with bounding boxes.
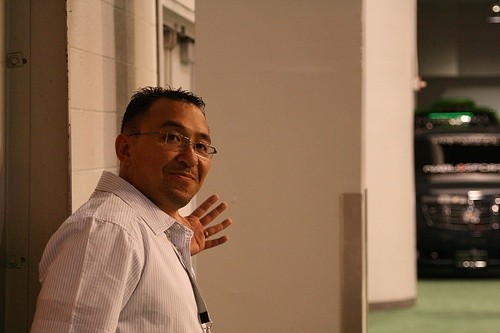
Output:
[131,129,217,160]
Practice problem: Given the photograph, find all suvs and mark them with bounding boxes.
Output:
[413,114,500,279]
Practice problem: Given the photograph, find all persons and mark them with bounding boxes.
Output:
[28,85,233,333]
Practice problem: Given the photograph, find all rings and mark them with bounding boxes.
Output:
[205,229,209,236]
